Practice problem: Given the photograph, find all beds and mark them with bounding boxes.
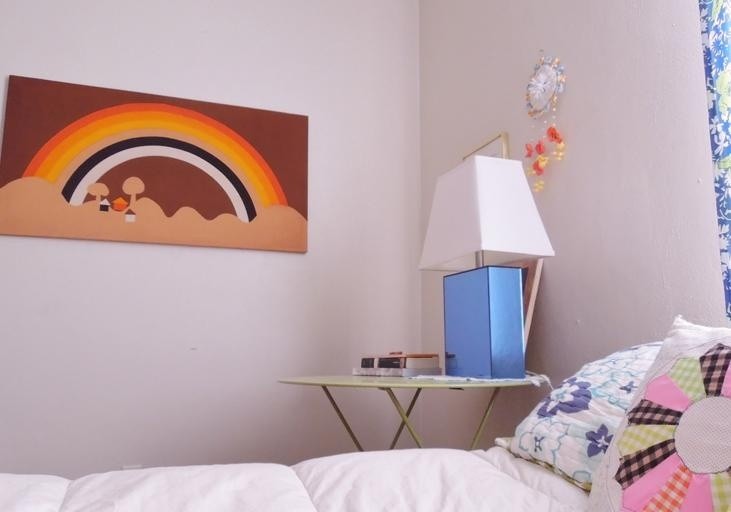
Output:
[0,437,591,512]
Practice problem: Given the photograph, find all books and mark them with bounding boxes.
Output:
[360,352,442,369]
[352,367,443,377]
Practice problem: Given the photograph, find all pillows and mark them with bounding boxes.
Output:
[585,315,731,512]
[504,342,662,489]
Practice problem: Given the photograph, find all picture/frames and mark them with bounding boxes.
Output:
[501,257,544,353]
[461,134,511,160]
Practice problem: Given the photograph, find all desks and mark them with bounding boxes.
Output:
[276,372,549,452]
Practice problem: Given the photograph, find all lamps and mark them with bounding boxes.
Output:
[418,155,558,380]
